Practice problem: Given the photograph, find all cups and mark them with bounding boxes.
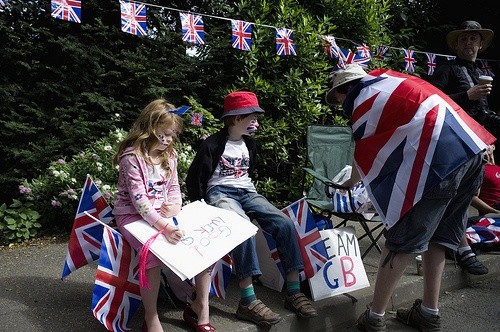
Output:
[478,75,493,86]
[416,255,423,276]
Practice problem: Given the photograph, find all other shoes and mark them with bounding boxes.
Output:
[455,249,488,275]
[183,305,216,332]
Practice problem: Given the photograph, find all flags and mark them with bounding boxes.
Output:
[261,199,329,282]
[62,177,120,280]
[403,50,415,72]
[276,28,296,55]
[91,226,142,332]
[375,46,388,61]
[320,35,371,66]
[480,60,495,76]
[427,53,436,75]
[51,0,81,23]
[179,13,204,44]
[120,0,147,36]
[190,252,233,299]
[231,20,252,51]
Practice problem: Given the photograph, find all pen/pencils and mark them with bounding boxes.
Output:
[172,216,185,238]
[162,183,169,203]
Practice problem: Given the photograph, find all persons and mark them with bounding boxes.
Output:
[472,164,500,215]
[431,21,494,275]
[186,91,317,325]
[112,100,217,332]
[325,64,496,332]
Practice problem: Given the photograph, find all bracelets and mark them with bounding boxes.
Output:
[138,223,169,288]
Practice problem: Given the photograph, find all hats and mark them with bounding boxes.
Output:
[445,21,493,52]
[326,64,367,105]
[219,91,266,119]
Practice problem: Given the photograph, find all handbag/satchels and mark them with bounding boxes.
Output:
[329,165,370,213]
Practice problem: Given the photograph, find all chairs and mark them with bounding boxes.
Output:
[300,124,386,263]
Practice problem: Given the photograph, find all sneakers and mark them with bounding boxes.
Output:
[358,308,386,332]
[284,294,317,319]
[236,299,282,325]
[395,299,441,331]
